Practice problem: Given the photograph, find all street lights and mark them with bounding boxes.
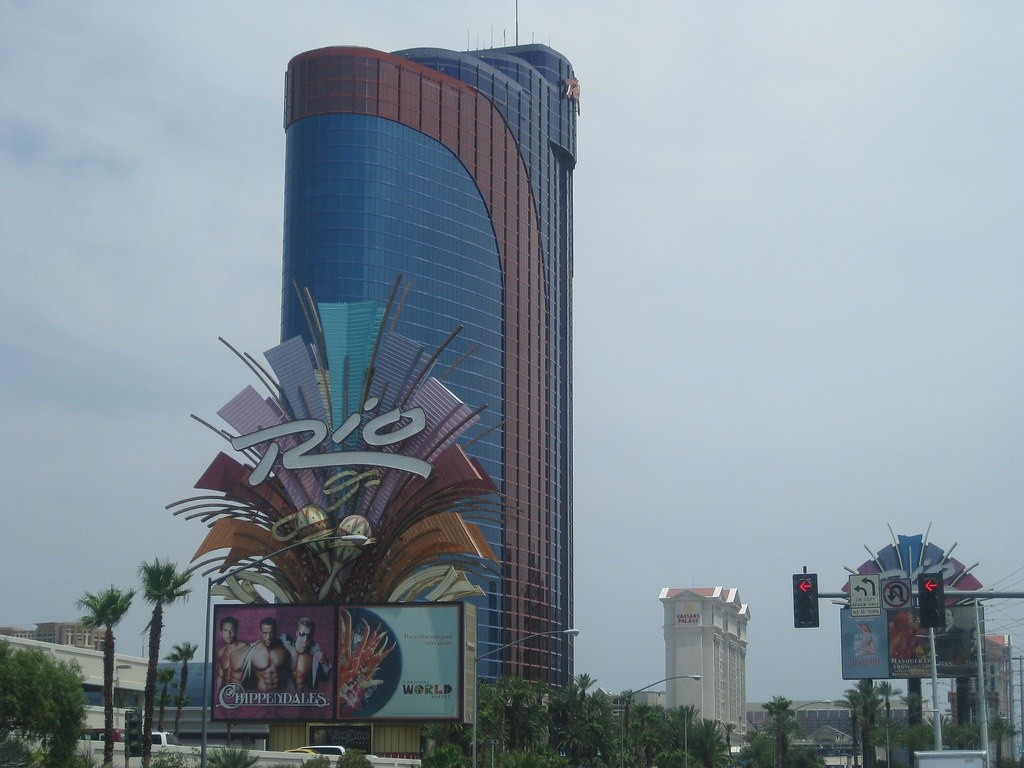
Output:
[471,629,580,768]
[621,674,702,768]
[772,699,831,768]
[198,534,370,768]
[885,708,939,768]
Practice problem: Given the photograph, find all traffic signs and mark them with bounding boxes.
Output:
[849,574,881,617]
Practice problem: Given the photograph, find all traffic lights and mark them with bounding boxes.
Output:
[917,572,947,628]
[792,573,820,629]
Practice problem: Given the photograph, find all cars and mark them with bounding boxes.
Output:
[284,749,322,754]
[77,728,121,742]
[122,731,183,746]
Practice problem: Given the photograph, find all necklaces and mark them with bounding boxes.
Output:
[294,643,306,655]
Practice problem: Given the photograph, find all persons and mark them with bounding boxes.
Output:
[937,607,970,660]
[214,617,249,689]
[888,610,919,658]
[244,617,290,690]
[287,617,332,691]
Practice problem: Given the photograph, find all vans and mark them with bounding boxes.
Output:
[301,744,348,755]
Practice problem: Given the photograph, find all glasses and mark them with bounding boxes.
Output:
[298,632,312,637]
